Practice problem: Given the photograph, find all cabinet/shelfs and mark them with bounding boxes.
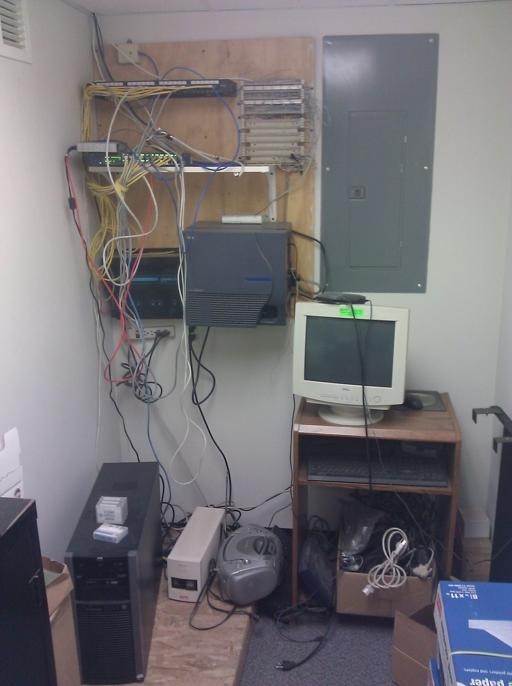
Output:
[289,387,465,626]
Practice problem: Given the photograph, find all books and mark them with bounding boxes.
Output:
[420,578,511,686]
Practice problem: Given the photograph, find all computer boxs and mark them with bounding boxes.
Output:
[63,463,163,682]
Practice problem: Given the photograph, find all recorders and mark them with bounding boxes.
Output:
[216,524,285,606]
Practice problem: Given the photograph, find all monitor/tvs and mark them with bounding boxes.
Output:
[293,303,409,426]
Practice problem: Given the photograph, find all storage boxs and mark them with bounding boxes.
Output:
[42,551,86,686]
[324,526,436,625]
[381,602,443,685]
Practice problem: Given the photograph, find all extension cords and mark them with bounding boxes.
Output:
[125,327,175,340]
